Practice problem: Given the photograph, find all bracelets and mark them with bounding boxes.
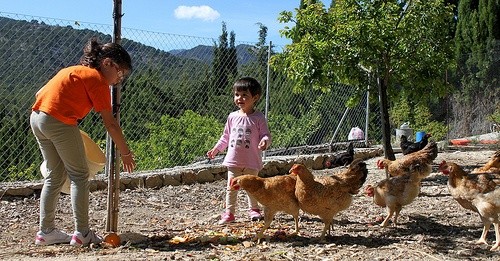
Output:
[121,151,131,156]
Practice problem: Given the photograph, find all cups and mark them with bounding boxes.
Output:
[395,128,414,143]
[416,131,425,142]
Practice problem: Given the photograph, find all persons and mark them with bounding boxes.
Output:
[30,38,135,246]
[207,77,271,225]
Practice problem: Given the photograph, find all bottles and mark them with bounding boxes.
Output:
[400,122,409,129]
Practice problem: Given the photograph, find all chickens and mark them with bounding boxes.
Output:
[230,143,368,245]
[364,132,438,228]
[438,150,500,251]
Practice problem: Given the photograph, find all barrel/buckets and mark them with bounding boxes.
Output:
[40,131,107,194]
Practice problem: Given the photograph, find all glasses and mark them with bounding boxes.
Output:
[111,60,126,80]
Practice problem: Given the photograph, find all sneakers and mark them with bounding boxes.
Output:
[218,212,235,225]
[35,228,74,246]
[70,229,103,247]
[249,209,262,220]
